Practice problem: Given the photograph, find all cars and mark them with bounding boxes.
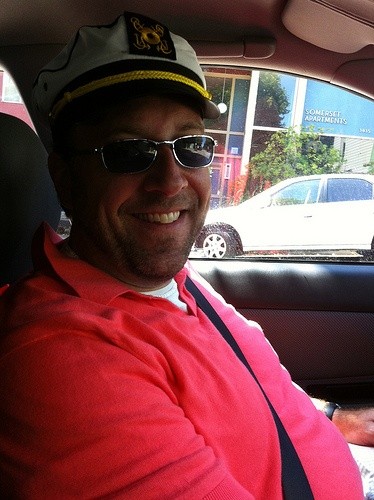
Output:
[192,172,373,263]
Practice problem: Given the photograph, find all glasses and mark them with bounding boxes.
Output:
[70,134,218,176]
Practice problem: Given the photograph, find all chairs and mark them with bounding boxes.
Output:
[0,113,63,368]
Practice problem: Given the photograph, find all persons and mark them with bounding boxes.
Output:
[1,19,372,500]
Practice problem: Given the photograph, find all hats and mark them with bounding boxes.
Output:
[29,11,223,127]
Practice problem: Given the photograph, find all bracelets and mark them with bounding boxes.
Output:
[325,402,336,420]
